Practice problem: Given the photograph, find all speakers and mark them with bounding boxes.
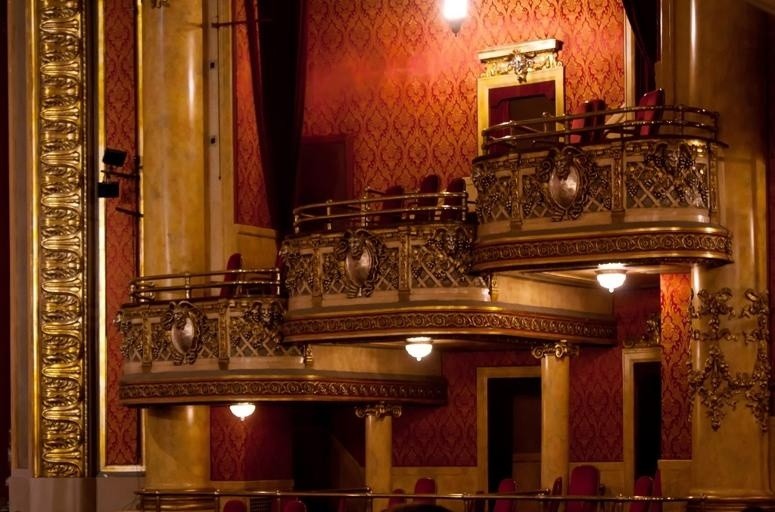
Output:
[103,148,127,167]
[98,182,119,197]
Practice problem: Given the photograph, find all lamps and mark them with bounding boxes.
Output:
[593,262,629,294]
[405,336,434,362]
[442,0,469,37]
[229,402,257,422]
[94,147,145,219]
